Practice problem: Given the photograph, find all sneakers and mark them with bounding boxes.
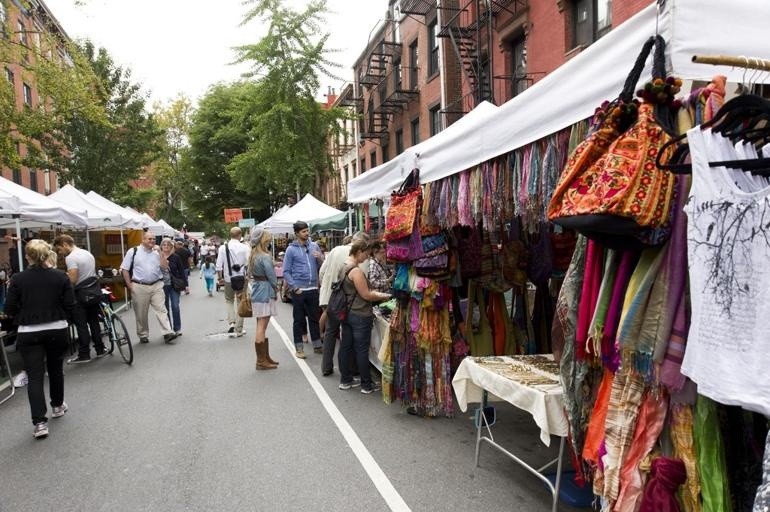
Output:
[32,422,48,439]
[140,338,148,342]
[164,331,182,343]
[67,356,91,364]
[361,382,381,394]
[339,378,360,389]
[52,403,67,419]
[228,322,246,336]
[296,351,306,358]
[97,347,109,357]
[13,372,29,388]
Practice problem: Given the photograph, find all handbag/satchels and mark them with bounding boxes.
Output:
[171,276,185,292]
[231,276,243,290]
[383,168,452,281]
[548,102,680,250]
[75,276,101,308]
[121,270,133,287]
[237,292,252,317]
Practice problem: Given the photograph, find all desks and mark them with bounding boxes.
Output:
[450,353,569,512]
[370,310,393,386]
[1,329,24,406]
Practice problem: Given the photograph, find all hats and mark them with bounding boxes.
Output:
[251,229,264,248]
[294,222,308,232]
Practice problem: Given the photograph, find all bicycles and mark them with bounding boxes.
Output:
[94,286,136,367]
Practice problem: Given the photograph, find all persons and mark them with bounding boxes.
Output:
[2,235,109,439]
[283,220,328,359]
[319,230,395,394]
[246,227,280,370]
[120,229,222,344]
[217,226,251,336]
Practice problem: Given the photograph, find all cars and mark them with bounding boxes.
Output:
[280,250,330,302]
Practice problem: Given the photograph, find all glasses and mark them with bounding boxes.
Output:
[304,244,310,253]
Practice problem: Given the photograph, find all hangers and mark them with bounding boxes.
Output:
[652,50,770,179]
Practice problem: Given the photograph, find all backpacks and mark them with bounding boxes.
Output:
[327,266,358,322]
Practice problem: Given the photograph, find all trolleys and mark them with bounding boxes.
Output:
[215,264,225,291]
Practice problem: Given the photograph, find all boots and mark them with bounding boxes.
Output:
[255,338,278,370]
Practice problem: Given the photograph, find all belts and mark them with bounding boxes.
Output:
[254,276,268,281]
[131,280,161,285]
